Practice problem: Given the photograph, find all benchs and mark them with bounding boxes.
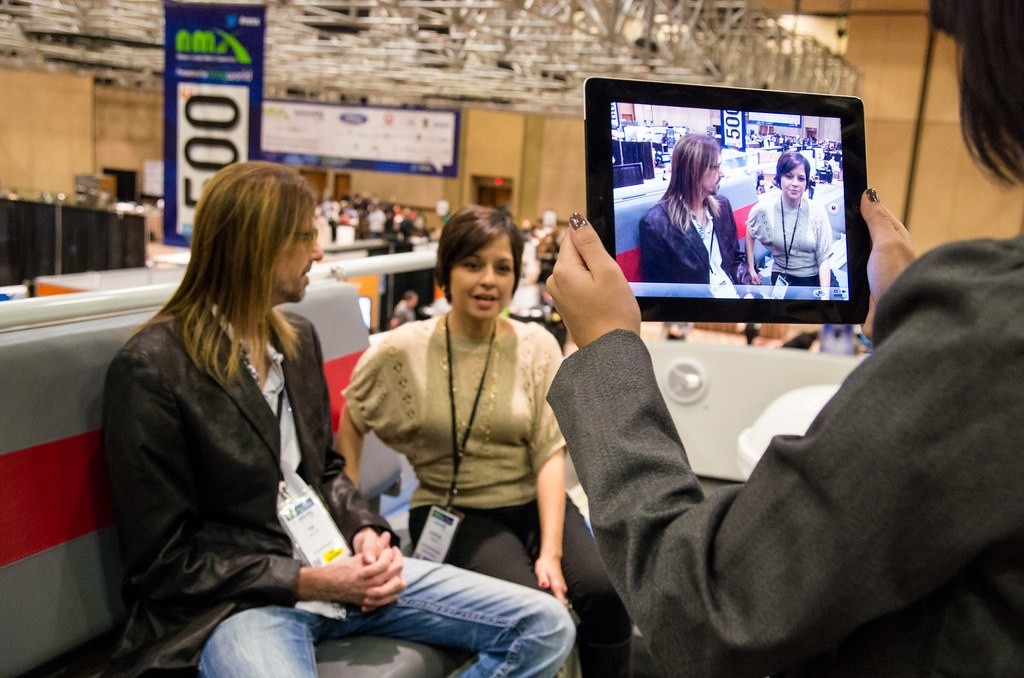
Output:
[0,283,475,678]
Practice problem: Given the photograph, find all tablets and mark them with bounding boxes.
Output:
[581,75,875,324]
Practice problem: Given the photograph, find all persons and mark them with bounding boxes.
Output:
[389,290,418,328]
[90,159,576,678]
[745,150,844,300]
[519,215,565,284]
[542,1,1024,678]
[320,189,432,244]
[638,134,763,300]
[333,197,633,678]
[736,323,762,346]
[660,322,694,341]
